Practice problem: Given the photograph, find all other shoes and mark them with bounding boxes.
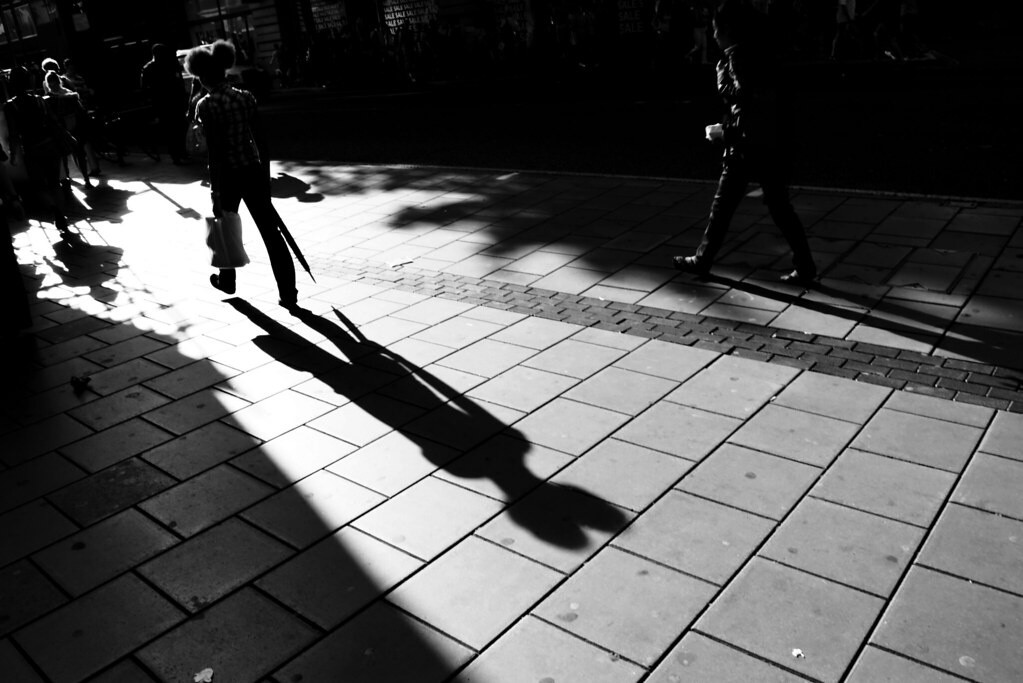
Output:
[779,269,817,283]
[674,256,710,276]
[173,160,183,165]
[210,270,237,294]
[279,298,297,306]
[56,220,68,231]
[183,156,193,160]
[88,168,101,176]
[86,181,94,189]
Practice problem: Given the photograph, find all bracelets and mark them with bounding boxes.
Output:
[10,192,23,202]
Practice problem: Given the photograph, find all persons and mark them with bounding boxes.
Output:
[135,36,310,313]
[541,0,952,66]
[671,0,819,285]
[0,55,118,333]
[292,11,525,83]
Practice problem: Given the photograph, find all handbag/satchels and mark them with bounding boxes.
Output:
[51,123,78,155]
[206,192,250,268]
